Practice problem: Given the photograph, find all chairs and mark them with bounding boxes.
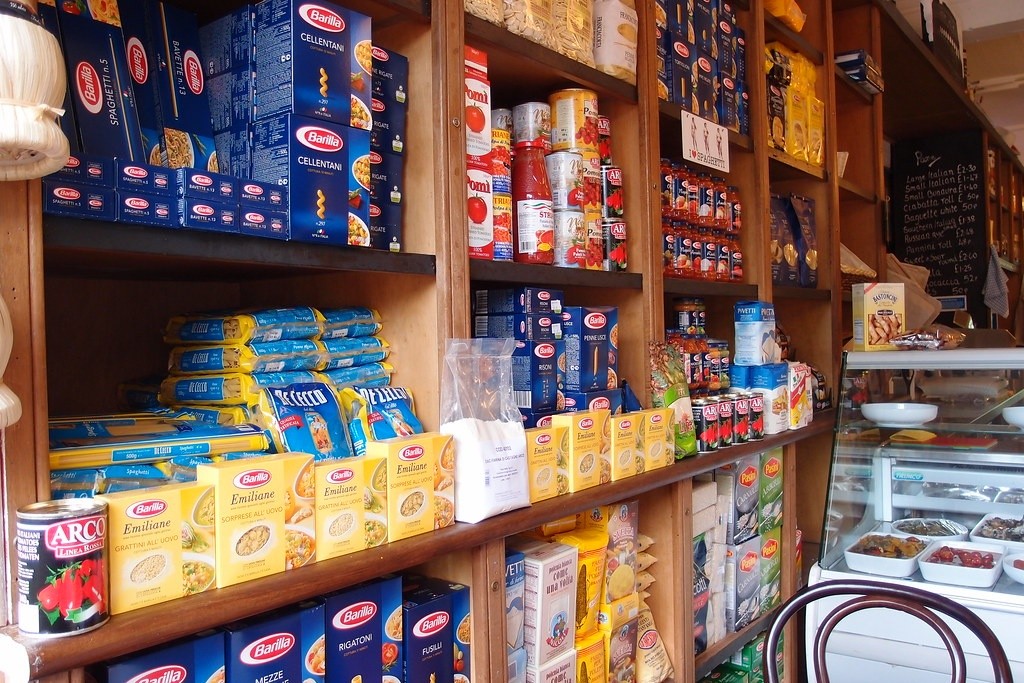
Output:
[762,579,1014,683]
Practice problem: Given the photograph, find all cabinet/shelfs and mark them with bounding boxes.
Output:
[989,135,1023,346]
[805,344,1024,683]
[0,0,886,682]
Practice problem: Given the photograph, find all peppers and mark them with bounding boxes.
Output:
[37,559,106,622]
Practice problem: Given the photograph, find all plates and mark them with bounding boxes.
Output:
[968,513,1024,549]
[890,518,968,541]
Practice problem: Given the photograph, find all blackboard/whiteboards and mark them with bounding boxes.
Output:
[890,125,989,308]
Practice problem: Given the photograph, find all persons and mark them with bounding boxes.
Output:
[703,122,710,156]
[715,128,724,159]
[690,117,699,151]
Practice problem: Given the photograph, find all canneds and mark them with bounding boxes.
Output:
[659,157,743,282]
[16,497,110,638]
[691,391,764,453]
[491,88,628,272]
[664,298,730,398]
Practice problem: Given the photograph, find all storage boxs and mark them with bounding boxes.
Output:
[38,0,882,683]
[851,283,905,352]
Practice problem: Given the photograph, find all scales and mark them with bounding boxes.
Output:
[915,294,1010,399]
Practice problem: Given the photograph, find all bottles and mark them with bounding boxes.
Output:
[514,141,554,264]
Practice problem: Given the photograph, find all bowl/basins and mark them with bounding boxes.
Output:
[845,531,934,578]
[1002,407,1024,431]
[919,541,1008,588]
[861,403,938,426]
[1003,553,1024,584]
